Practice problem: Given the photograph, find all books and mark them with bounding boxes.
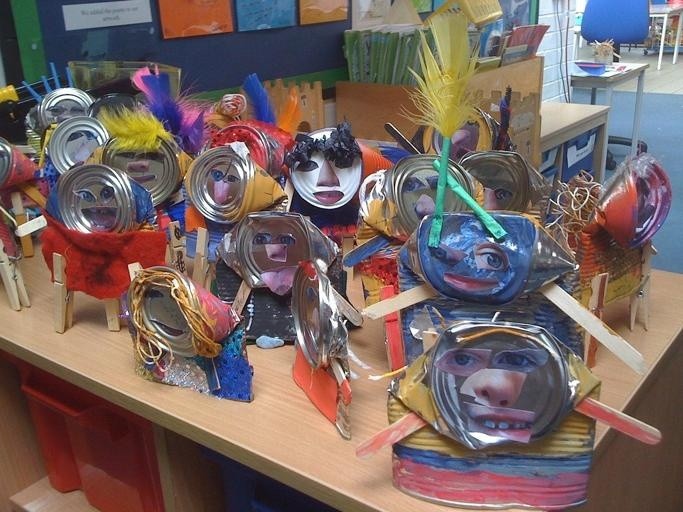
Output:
[344,23,437,85]
[475,19,550,75]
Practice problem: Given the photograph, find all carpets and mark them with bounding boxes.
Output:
[541,89,683,274]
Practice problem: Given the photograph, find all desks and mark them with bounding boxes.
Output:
[569,61,649,162]
[538,101,609,194]
[647,3,683,70]
[0,139,682,512]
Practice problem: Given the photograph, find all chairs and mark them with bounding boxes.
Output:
[580,0,649,171]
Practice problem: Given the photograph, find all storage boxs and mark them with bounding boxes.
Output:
[334,55,543,181]
